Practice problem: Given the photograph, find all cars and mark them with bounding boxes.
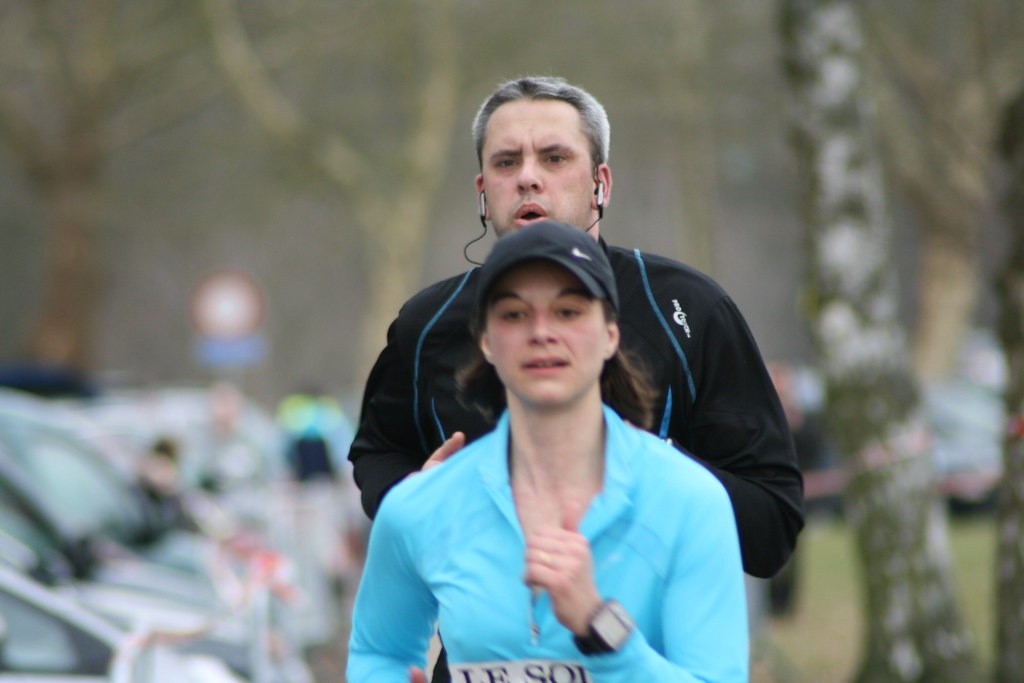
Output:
[0,360,367,682]
[761,326,1014,524]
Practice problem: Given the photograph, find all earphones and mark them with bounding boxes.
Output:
[480,190,487,220]
[594,182,604,219]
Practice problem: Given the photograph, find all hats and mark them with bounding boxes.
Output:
[473,219,619,334]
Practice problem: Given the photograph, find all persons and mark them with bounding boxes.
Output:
[344,221,753,682]
[1,316,363,683]
[347,76,803,578]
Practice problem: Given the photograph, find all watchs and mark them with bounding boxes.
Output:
[571,599,636,655]
